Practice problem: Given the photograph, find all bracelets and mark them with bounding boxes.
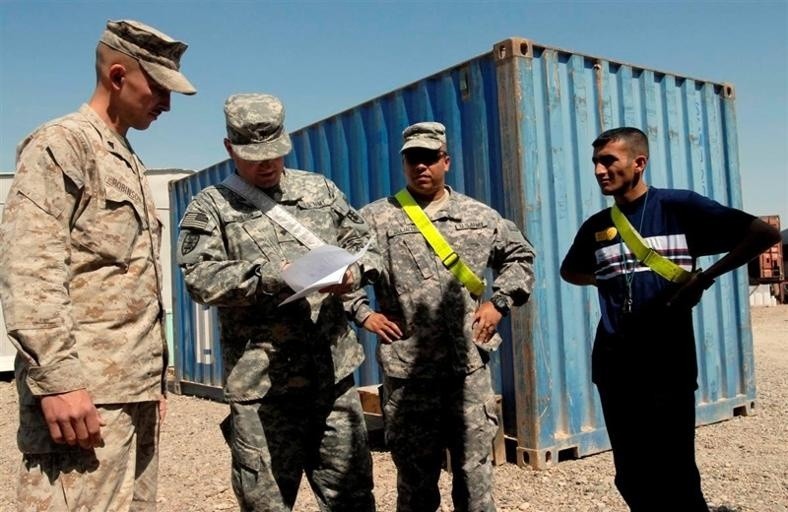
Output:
[693,266,715,290]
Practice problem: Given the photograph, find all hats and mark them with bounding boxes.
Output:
[100,20,197,95]
[224,94,292,161]
[399,122,447,154]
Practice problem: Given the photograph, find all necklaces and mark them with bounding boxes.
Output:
[615,186,649,313]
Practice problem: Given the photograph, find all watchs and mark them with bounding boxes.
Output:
[489,293,512,316]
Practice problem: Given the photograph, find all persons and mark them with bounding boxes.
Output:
[341,120,536,512]
[558,125,781,512]
[0,18,196,510]
[175,90,383,512]
